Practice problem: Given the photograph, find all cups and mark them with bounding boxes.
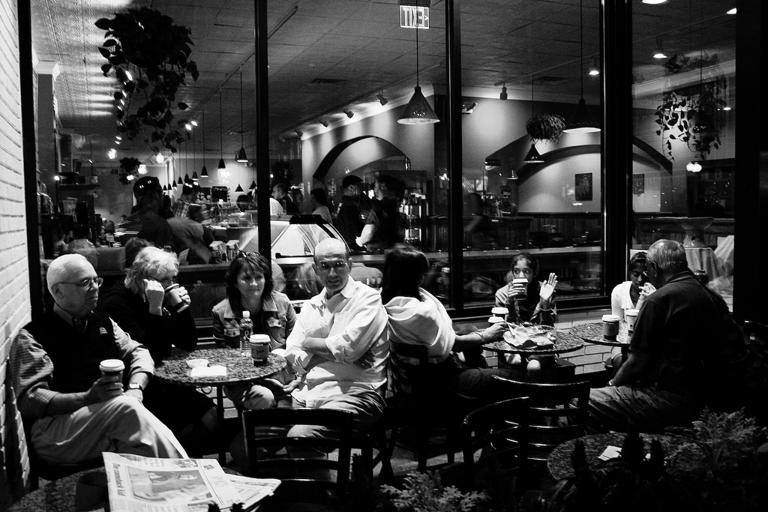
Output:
[602,314,619,340]
[489,317,505,327]
[440,267,451,285]
[512,278,528,301]
[626,314,637,336]
[250,333,270,365]
[163,284,190,313]
[100,359,125,384]
[491,307,508,322]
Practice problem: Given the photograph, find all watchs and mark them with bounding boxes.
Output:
[126,382,144,393]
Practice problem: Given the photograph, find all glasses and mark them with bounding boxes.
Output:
[317,262,346,272]
[146,275,180,290]
[50,277,103,291]
[642,265,654,277]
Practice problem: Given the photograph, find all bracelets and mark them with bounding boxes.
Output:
[475,331,485,343]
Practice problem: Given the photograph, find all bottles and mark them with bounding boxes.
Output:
[239,311,253,356]
[215,243,239,264]
[89,228,92,241]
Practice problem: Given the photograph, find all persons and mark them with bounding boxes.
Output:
[209,252,292,426]
[40,174,515,276]
[9,254,190,466]
[105,246,224,462]
[564,238,728,435]
[610,251,660,344]
[493,255,558,387]
[377,246,548,458]
[229,238,390,491]
